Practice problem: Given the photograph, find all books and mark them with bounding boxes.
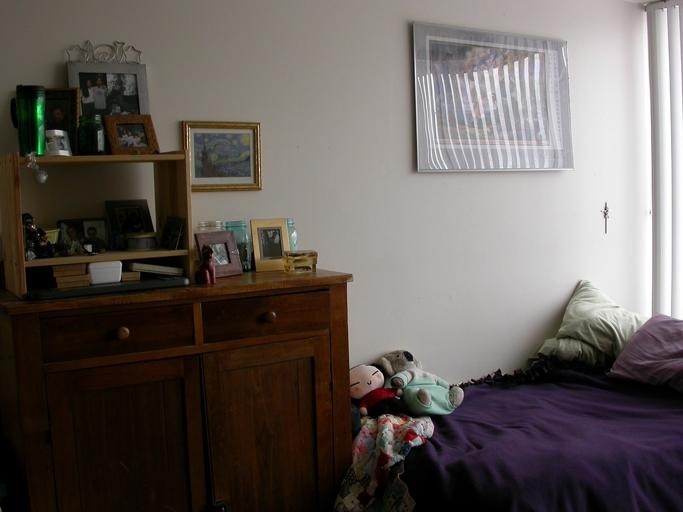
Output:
[52,262,184,288]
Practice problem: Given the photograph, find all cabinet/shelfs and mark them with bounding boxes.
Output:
[0,151,191,297]
[1,270,356,510]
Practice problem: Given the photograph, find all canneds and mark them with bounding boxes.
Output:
[78,114,107,155]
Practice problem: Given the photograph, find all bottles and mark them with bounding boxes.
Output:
[198,216,297,273]
[9,83,48,158]
[77,113,104,153]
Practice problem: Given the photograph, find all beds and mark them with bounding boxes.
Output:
[352,356,683,510]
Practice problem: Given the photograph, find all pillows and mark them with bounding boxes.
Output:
[610,312,683,392]
[538,337,601,366]
[557,280,645,359]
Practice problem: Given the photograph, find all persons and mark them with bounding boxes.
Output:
[48,78,145,146]
[84,227,105,246]
[66,226,79,241]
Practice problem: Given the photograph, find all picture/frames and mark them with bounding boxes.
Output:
[249,218,292,271]
[70,60,150,136]
[44,88,81,150]
[181,119,261,193]
[104,198,154,233]
[196,230,245,276]
[410,21,576,171]
[162,216,184,249]
[104,113,161,154]
